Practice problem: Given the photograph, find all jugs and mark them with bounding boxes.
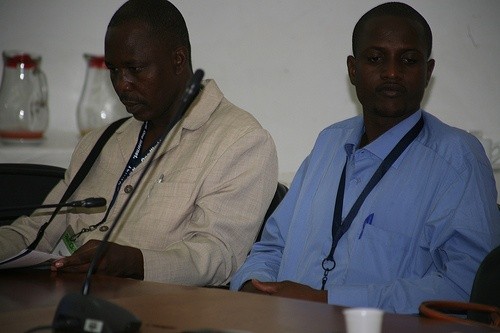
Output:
[76,51,134,133]
[1,51,52,140]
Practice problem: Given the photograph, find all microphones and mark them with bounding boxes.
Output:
[51,69,205,333]
[0,197,107,211]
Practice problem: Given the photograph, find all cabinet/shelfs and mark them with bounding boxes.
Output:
[0,271,500,333]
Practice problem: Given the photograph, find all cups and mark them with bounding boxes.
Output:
[344,307,384,332]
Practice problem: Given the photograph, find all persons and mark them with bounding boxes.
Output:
[229,0,500,314]
[0,0,280,287]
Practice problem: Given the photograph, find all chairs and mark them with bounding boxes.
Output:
[0,163,65,227]
[466,245,500,325]
